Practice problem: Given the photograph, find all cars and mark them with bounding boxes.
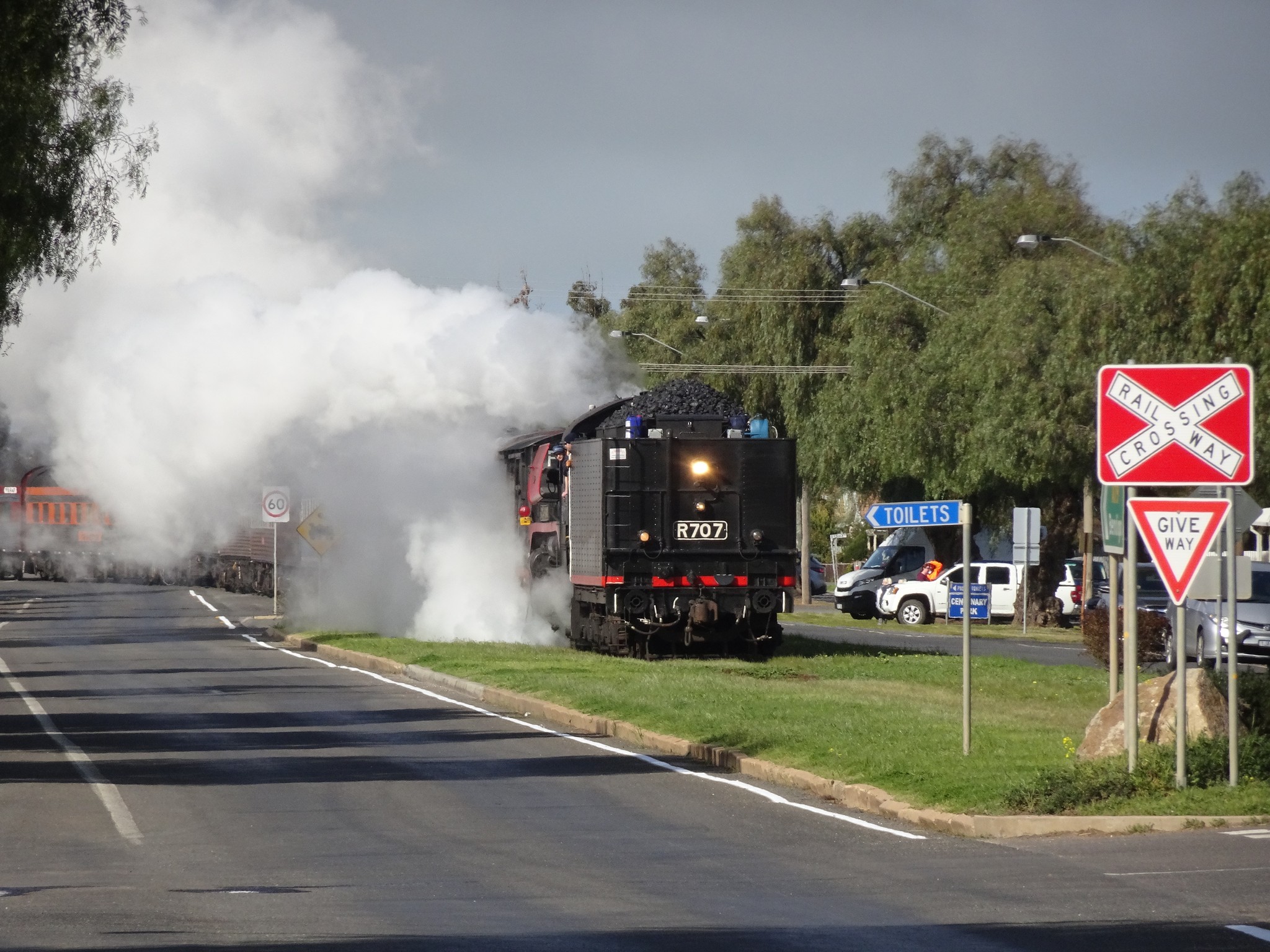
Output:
[1094,561,1170,618]
[1054,560,1106,621]
[1161,561,1270,670]
[794,554,827,597]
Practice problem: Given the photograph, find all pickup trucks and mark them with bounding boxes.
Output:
[877,561,1025,624]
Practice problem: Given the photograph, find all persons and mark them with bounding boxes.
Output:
[881,550,890,562]
[877,577,892,625]
[898,578,907,584]
[917,561,943,582]
[549,442,572,499]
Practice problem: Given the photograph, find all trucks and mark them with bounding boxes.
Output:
[833,528,935,619]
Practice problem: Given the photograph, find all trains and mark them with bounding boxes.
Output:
[497,378,805,661]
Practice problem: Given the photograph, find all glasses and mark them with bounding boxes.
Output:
[564,443,567,445]
[555,452,562,457]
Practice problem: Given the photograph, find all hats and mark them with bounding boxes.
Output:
[552,445,564,454]
[564,432,579,444]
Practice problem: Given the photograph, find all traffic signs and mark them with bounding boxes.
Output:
[864,497,963,530]
